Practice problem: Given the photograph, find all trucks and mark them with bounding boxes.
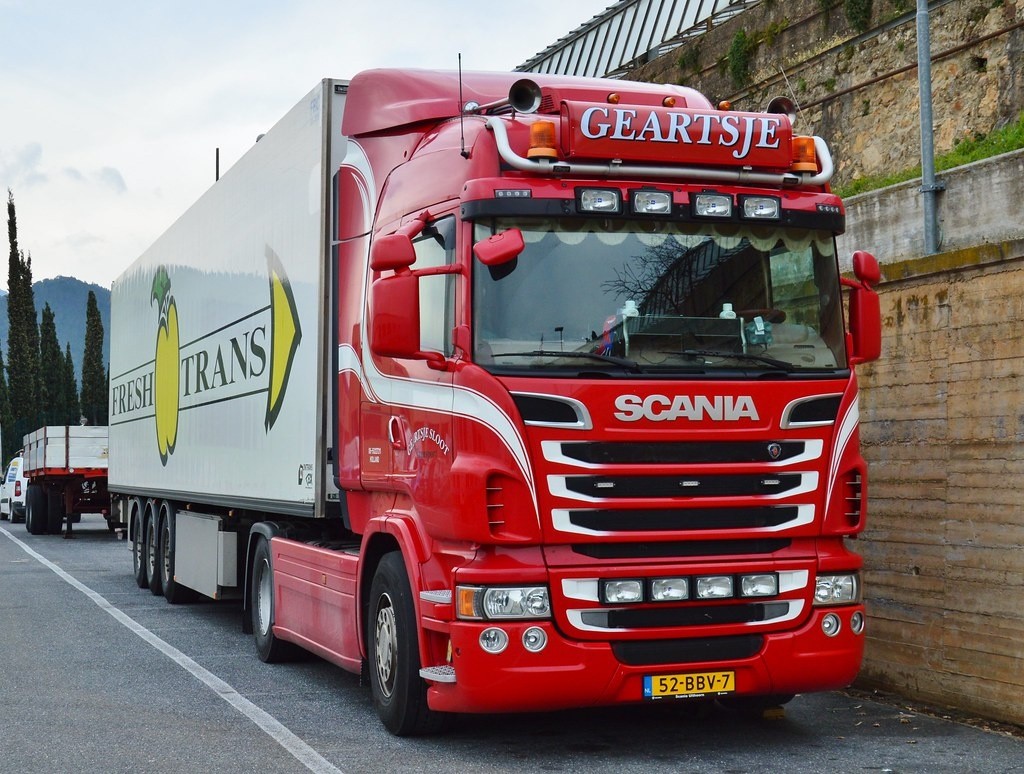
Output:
[16,425,121,536]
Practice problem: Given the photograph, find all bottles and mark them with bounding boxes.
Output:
[623,300,639,316]
[719,303,736,318]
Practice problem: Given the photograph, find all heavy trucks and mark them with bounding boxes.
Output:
[108,67,883,738]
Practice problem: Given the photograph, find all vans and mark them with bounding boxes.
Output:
[1,456,26,524]
